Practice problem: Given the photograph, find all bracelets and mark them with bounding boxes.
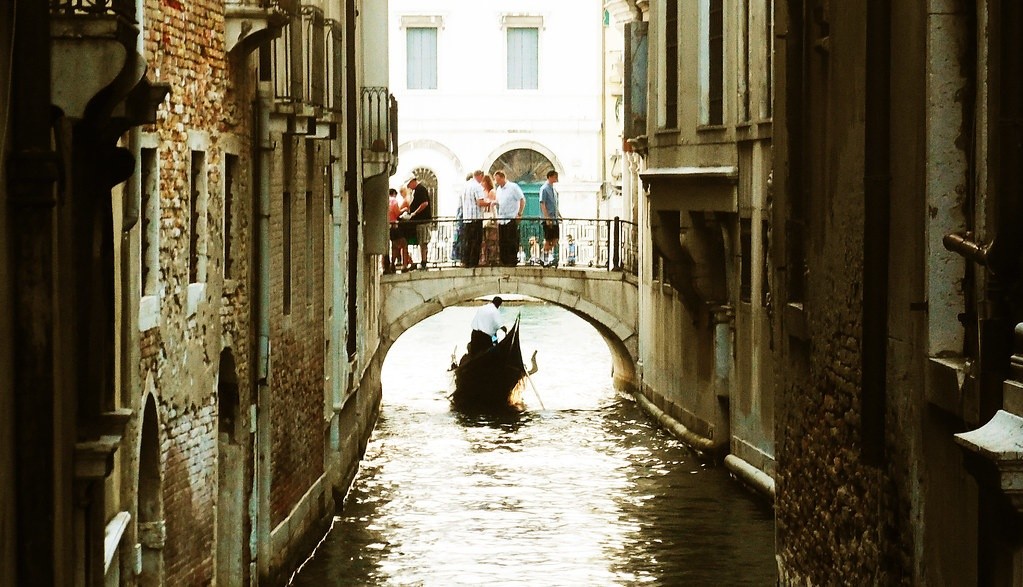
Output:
[517,212,522,216]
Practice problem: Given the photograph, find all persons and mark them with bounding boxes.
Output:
[450,170,525,267]
[566,235,575,265]
[382,176,432,272]
[471,297,508,357]
[517,171,561,264]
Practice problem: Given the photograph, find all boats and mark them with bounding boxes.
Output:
[451,311,530,414]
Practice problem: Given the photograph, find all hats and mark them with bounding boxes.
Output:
[403,173,415,185]
